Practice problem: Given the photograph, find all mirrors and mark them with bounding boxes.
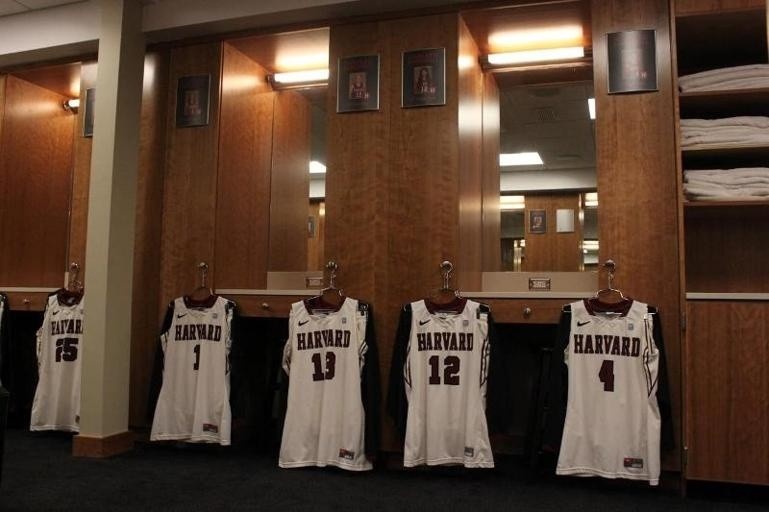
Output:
[268,84,327,271]
[482,60,599,273]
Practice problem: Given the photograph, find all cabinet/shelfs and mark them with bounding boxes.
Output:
[667,0,768,502]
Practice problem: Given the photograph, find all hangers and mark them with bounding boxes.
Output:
[64,262,83,293]
[559,261,660,316]
[305,261,369,312]
[167,263,237,311]
[404,259,491,315]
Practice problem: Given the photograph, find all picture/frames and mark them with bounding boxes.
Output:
[308,215,318,239]
[82,87,93,138]
[335,47,446,114]
[527,208,548,234]
[604,29,660,95]
[172,72,211,130]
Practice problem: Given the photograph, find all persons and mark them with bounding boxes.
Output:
[413,68,433,93]
[185,89,201,117]
[349,71,367,99]
